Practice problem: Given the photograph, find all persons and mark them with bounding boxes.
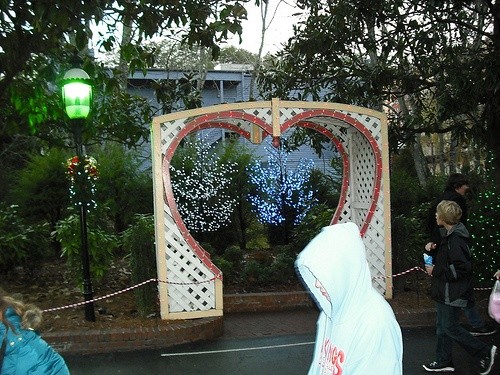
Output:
[427,172,498,336]
[0,286,71,375]
[423,201,497,375]
[293,222,404,375]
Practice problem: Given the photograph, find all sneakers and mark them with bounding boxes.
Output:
[478,344,498,375]
[469,325,497,336]
[422,360,457,373]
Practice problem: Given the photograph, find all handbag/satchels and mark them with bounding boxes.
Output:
[488,269,500,323]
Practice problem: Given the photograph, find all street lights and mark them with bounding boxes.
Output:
[62,67,95,323]
